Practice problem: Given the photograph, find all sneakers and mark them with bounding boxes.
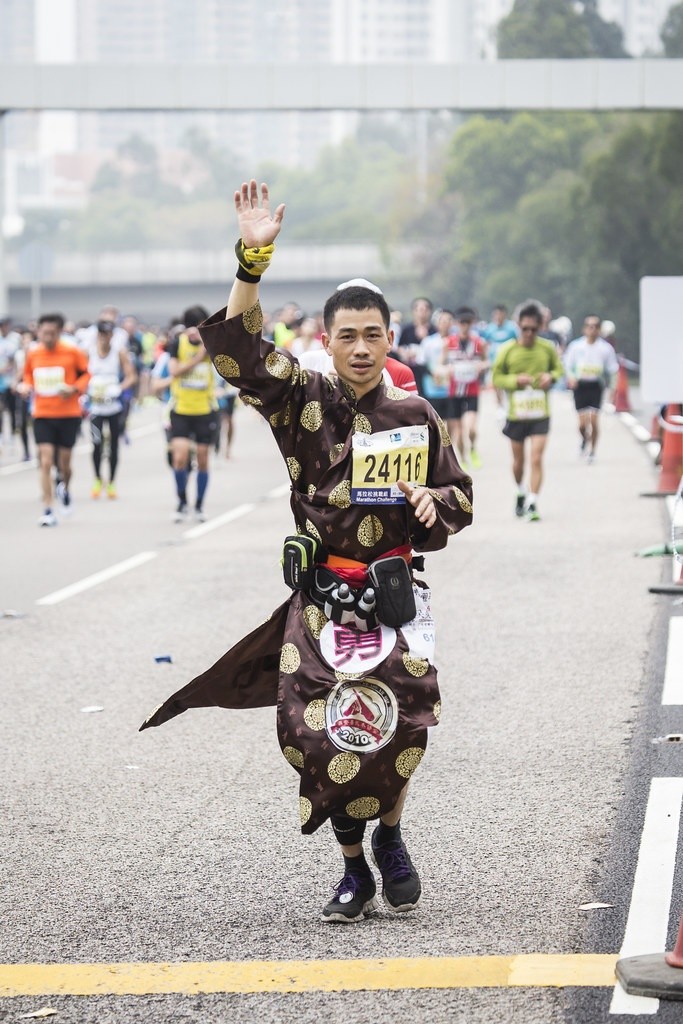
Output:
[369,825,422,912]
[321,867,381,922]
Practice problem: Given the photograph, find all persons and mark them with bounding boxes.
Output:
[193,180,473,921]
[1,278,683,523]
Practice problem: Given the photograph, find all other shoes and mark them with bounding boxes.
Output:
[195,503,207,521]
[471,447,481,468]
[107,482,115,498]
[528,504,539,520]
[172,503,188,522]
[516,492,526,516]
[38,508,58,527]
[91,479,102,497]
[55,477,70,505]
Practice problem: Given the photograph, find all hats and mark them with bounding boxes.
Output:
[97,321,116,333]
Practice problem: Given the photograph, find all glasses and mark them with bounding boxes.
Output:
[522,325,537,332]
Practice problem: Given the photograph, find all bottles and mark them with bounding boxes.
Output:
[324,583,355,624]
[355,588,381,631]
